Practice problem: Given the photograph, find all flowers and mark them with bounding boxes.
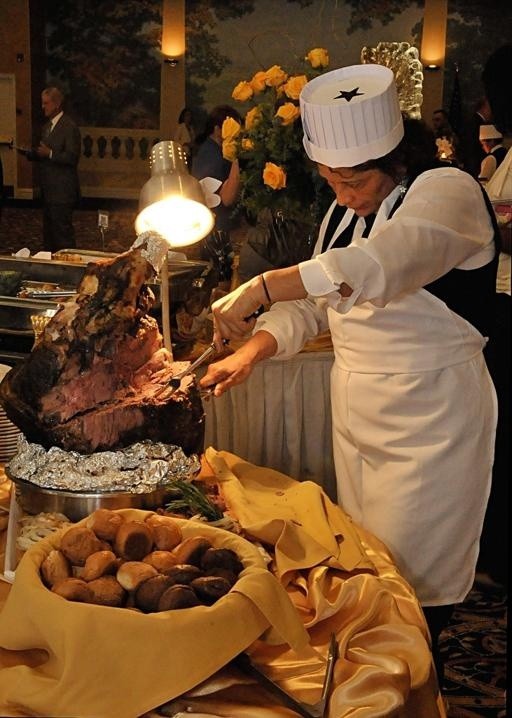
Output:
[222,48,336,264]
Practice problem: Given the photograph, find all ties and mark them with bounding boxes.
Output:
[43,121,52,138]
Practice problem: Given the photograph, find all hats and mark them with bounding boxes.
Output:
[299,64,404,168]
[478,125,502,140]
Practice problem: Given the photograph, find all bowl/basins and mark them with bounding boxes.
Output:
[5,459,202,519]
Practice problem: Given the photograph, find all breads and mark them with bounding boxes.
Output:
[42,507,244,614]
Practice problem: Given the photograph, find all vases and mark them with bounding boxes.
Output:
[266,220,313,268]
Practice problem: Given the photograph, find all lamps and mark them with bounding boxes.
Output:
[164,58,178,70]
[425,64,440,73]
[133,141,215,362]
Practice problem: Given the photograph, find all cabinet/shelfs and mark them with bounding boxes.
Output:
[0,249,207,363]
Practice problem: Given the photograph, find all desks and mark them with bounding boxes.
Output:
[0,451,447,718]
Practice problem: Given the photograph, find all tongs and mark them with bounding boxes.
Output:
[235,632,339,716]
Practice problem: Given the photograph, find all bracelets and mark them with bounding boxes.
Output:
[260,273,272,305]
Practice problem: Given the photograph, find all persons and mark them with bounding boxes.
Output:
[199,64,501,647]
[428,97,511,295]
[28,87,80,250]
[175,103,242,282]
